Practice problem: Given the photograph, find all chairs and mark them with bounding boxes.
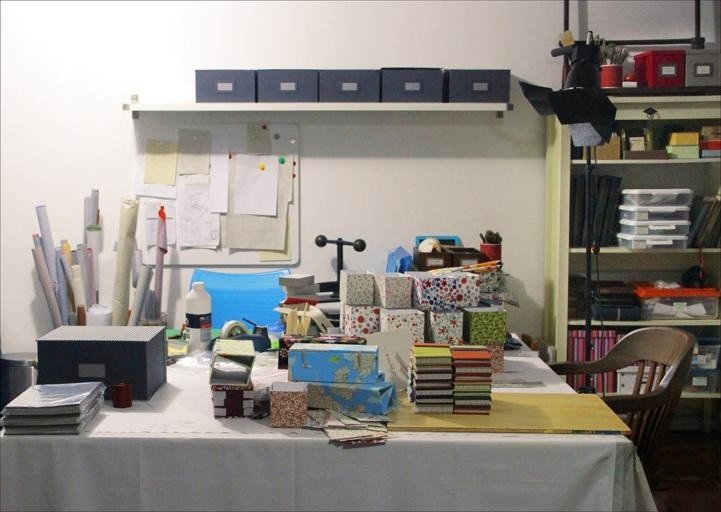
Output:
[548,326,699,452]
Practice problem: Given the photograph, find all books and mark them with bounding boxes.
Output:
[3,382,105,437]
[449,345,493,415]
[409,344,452,415]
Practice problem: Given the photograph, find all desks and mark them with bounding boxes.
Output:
[0,334,634,512]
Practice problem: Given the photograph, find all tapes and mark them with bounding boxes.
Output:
[220,320,248,338]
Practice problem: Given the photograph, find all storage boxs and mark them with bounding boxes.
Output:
[378,66,448,104]
[618,233,688,250]
[380,307,425,343]
[256,68,320,103]
[375,273,412,309]
[617,366,669,395]
[344,307,380,336]
[340,269,374,306]
[622,188,693,206]
[464,307,506,344]
[194,68,257,104]
[317,68,382,105]
[687,369,719,394]
[434,271,480,308]
[569,328,615,393]
[618,206,691,222]
[444,67,514,104]
[405,271,456,312]
[682,47,721,91]
[35,324,167,402]
[688,339,721,370]
[426,310,462,345]
[483,344,505,373]
[620,219,691,236]
[628,282,719,322]
[634,49,686,90]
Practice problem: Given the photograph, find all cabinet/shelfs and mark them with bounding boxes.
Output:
[120,102,517,121]
[544,93,721,474]
